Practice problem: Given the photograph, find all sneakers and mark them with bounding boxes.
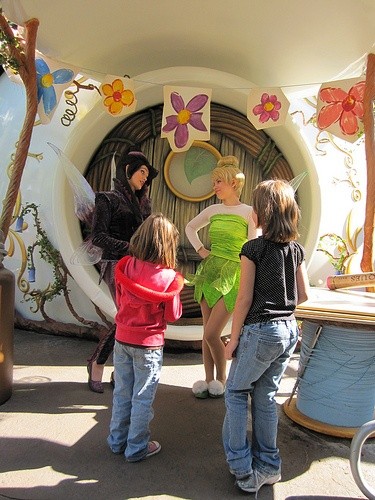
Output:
[144,441,161,458]
[236,467,282,500]
[232,469,253,478]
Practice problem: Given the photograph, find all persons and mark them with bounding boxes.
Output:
[218,177,308,494]
[107,211,184,462]
[184,156,260,401]
[88,151,159,394]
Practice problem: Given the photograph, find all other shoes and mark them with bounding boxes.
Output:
[86,361,104,393]
[191,381,210,398]
[112,371,117,387]
[208,379,226,398]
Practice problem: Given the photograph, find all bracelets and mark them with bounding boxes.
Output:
[195,244,203,254]
